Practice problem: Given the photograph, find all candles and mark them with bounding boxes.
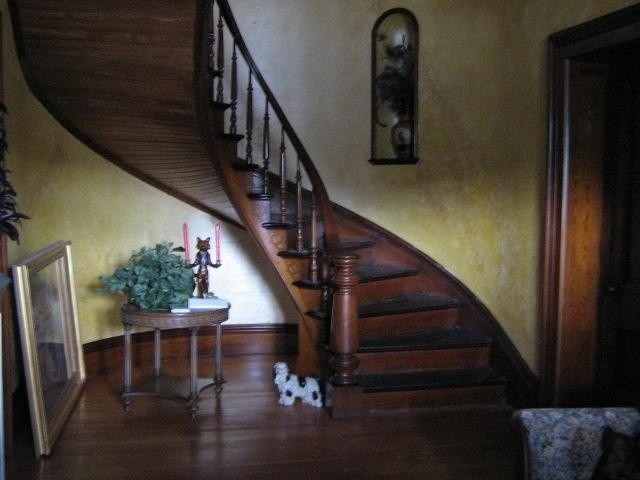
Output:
[183,222,190,260]
[215,223,221,259]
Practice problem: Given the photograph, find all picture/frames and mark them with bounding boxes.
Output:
[11,239,89,459]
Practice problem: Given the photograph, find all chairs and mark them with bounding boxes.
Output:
[509,405,639,480]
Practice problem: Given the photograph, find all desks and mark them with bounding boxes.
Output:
[119,298,229,423]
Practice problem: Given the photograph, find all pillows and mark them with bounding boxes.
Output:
[591,425,639,480]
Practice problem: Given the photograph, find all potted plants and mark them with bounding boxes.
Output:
[374,32,413,159]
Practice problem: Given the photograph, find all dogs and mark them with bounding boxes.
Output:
[273,361,323,409]
